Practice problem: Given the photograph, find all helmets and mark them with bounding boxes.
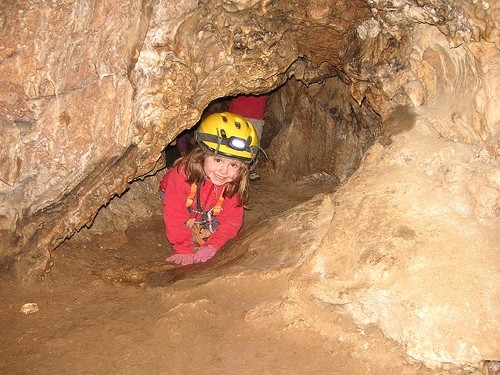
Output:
[194,111,260,162]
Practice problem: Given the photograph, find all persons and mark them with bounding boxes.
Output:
[163,111,259,268]
[221,95,270,180]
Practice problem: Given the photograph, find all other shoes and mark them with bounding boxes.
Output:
[248,169,260,181]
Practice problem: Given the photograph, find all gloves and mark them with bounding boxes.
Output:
[165,252,195,265]
[194,244,217,263]
